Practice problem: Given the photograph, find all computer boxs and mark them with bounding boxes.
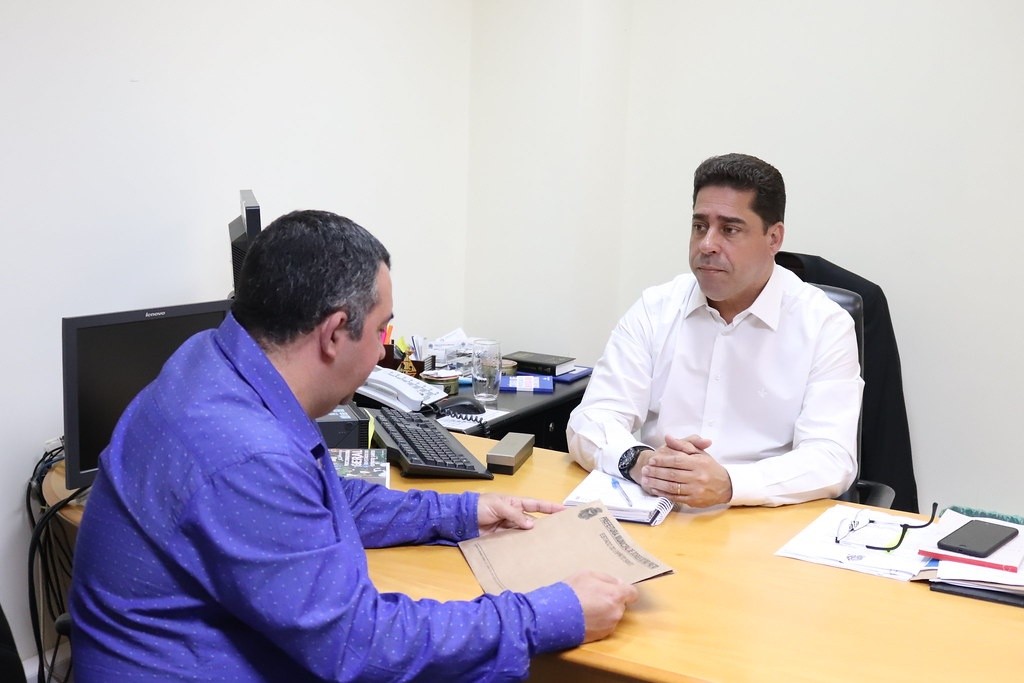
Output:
[310,401,370,450]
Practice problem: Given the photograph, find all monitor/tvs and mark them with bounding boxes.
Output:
[229,190,261,294]
[61,298,235,490]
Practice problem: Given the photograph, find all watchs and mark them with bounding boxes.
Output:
[618,446,653,485]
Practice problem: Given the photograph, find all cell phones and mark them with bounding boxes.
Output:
[937,520,1018,559]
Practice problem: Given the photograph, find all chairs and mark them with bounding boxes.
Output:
[808,283,863,504]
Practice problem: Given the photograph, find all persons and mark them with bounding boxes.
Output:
[565,154,864,507]
[66,209,641,683]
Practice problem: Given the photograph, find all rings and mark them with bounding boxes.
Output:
[678,484,681,495]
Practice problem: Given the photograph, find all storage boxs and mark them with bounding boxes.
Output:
[486,433,534,476]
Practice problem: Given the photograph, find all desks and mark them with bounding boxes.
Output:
[46,431,1024,683]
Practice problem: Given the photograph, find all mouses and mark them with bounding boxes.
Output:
[434,396,486,415]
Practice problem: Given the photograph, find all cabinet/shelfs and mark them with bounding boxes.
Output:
[431,373,594,453]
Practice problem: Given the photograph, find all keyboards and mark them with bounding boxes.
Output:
[359,407,495,481]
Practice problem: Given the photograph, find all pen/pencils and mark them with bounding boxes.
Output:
[611,477,633,507]
[380,323,393,344]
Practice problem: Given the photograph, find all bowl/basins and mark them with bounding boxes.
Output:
[482,359,517,377]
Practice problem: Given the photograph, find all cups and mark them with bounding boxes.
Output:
[446,346,474,378]
[472,339,499,402]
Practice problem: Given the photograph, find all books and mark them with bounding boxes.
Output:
[562,470,674,527]
[499,376,553,393]
[503,351,594,384]
[918,509,1024,607]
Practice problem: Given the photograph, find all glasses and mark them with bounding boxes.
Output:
[835,502,938,551]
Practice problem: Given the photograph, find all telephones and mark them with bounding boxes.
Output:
[352,364,448,413]
